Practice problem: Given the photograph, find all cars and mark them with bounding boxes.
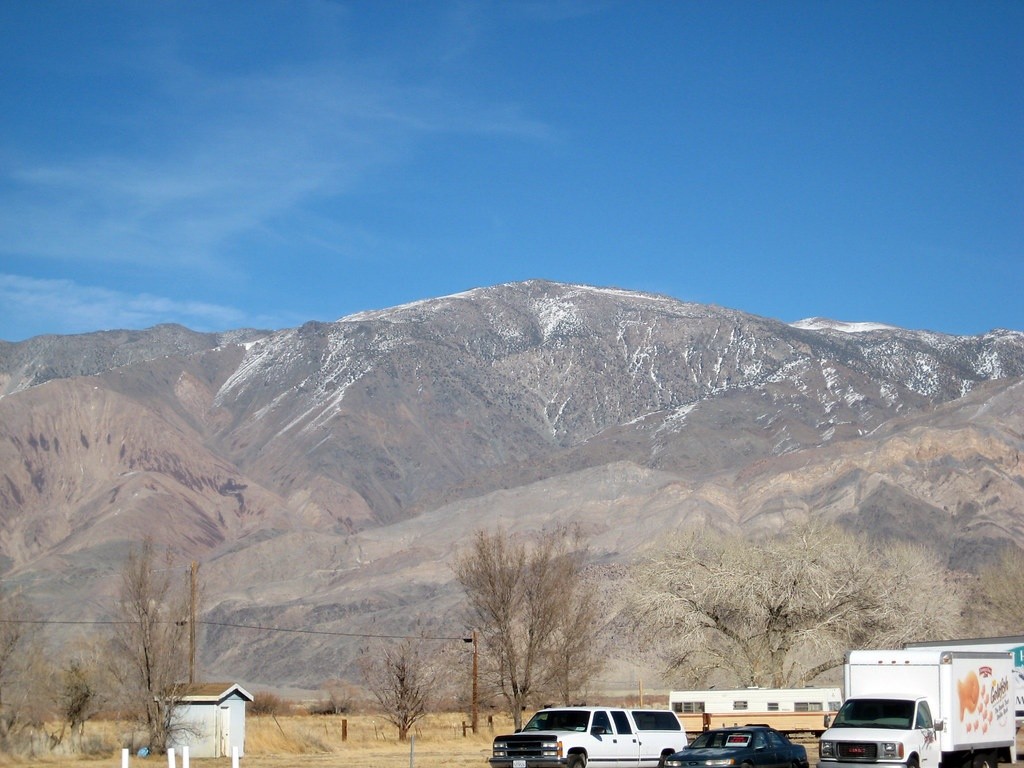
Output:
[663,726,810,768]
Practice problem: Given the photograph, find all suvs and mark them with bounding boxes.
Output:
[485,706,689,768]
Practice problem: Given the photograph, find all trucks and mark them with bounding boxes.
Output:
[815,647,1018,768]
